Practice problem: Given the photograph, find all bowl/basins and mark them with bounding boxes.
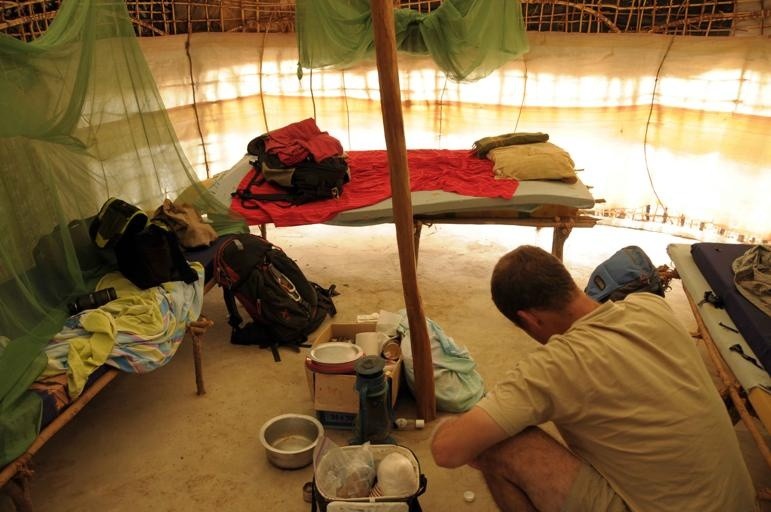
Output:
[310,343,362,367]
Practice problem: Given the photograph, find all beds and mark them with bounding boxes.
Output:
[666,241,770,476]
[176,133,604,275]
[1,212,248,509]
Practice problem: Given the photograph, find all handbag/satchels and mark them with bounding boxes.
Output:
[150,198,217,250]
[398,312,484,413]
[240,135,351,206]
[584,246,665,303]
[304,443,427,512]
[89,197,169,266]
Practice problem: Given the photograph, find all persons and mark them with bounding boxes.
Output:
[429,243,759,512]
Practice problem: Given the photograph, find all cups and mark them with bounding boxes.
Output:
[355,332,382,357]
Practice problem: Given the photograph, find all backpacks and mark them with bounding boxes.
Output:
[214,234,340,361]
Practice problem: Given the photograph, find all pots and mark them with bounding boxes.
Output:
[258,414,325,469]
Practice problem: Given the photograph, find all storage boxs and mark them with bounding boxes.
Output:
[304,320,404,427]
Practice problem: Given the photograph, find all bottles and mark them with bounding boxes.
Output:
[302,482,312,503]
[394,418,425,432]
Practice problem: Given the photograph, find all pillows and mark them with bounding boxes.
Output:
[486,140,575,181]
